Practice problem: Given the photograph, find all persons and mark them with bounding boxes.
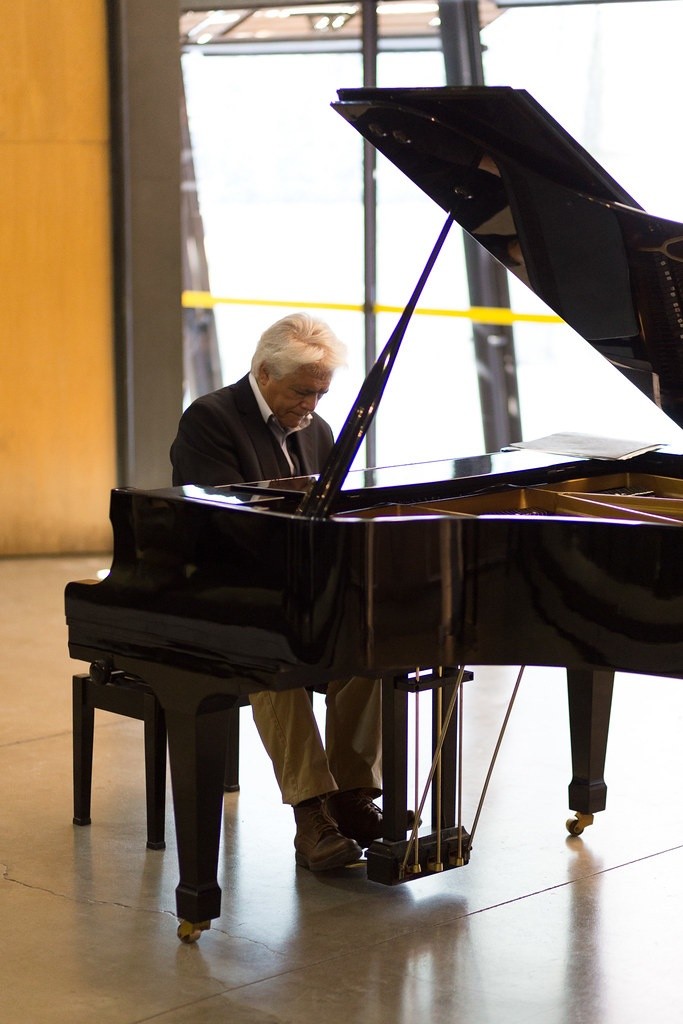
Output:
[171,312,424,875]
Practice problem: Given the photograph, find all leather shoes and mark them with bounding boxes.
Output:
[293,802,364,872]
[326,795,421,849]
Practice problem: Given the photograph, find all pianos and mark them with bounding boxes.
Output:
[64,82,682,943]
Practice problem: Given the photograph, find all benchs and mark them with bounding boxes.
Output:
[72,670,316,850]
